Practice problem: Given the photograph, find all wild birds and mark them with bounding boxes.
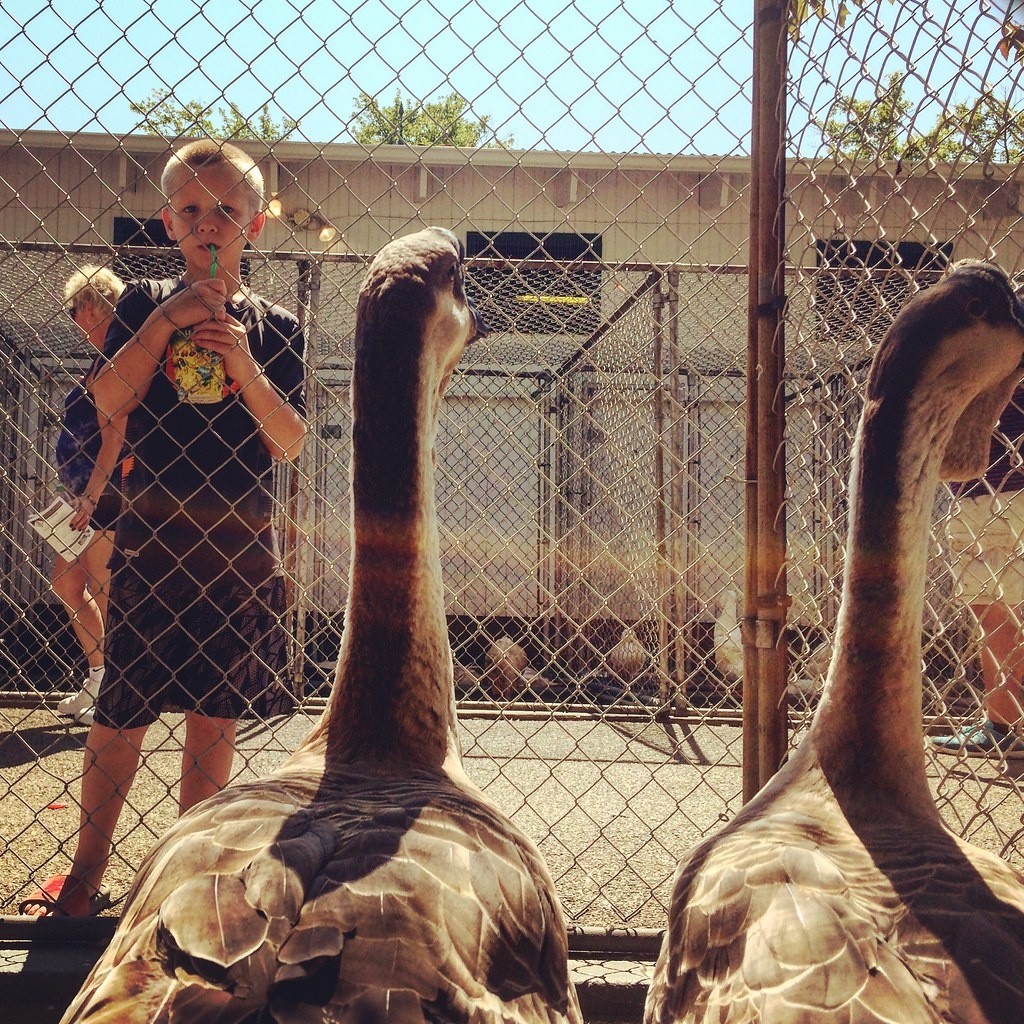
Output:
[645,259,1024,1024]
[51,225,585,1024]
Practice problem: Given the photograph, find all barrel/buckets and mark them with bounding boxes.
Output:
[970,605,1024,726]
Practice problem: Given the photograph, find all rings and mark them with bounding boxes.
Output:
[85,515,88,519]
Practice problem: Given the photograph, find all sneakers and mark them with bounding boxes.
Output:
[75,707,97,726]
[57,678,101,715]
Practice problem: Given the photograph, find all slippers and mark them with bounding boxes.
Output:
[18,874,111,916]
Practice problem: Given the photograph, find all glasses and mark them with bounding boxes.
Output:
[69,307,82,318]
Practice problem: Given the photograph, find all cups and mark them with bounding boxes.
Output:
[171,325,224,403]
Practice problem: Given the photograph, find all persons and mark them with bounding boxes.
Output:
[50,265,124,727]
[932,384,1024,758]
[19,138,305,919]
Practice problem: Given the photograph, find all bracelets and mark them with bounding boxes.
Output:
[81,493,97,511]
